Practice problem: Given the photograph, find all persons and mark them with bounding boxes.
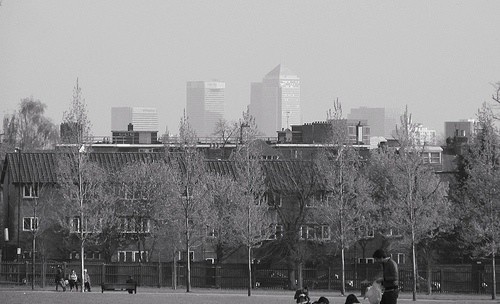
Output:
[83,269,91,292]
[126,276,133,294]
[345,294,362,304]
[69,269,78,292]
[55,268,66,291]
[294,286,329,304]
[373,249,399,304]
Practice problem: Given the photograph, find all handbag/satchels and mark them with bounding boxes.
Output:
[364,280,383,304]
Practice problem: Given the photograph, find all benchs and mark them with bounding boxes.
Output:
[102,283,136,294]
[56,282,86,292]
